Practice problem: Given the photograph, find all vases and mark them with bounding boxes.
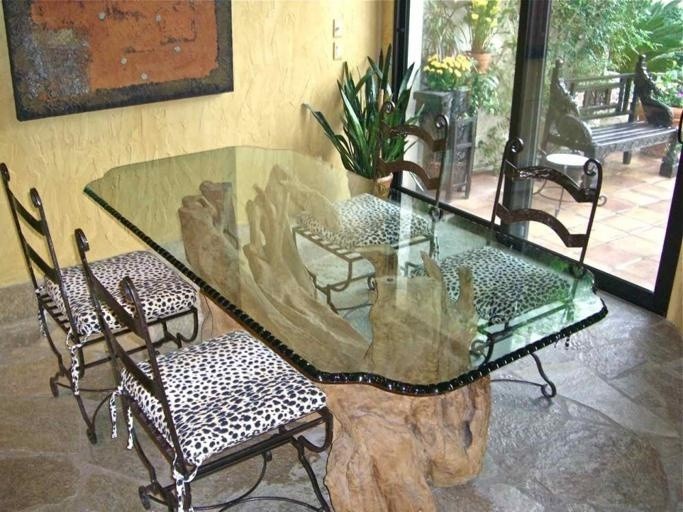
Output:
[636,104,683,158]
[464,51,500,82]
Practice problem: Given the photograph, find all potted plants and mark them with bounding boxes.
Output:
[301,42,431,200]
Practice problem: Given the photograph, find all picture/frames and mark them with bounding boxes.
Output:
[1,0,233,123]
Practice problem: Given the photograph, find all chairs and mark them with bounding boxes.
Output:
[0,159,201,443]
[407,134,604,398]
[292,103,454,314]
[62,223,332,512]
[542,55,682,177]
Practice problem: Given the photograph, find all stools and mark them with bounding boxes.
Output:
[532,154,603,218]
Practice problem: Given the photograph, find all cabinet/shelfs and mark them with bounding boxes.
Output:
[413,86,480,201]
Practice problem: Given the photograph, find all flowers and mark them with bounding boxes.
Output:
[461,1,516,52]
[428,54,472,89]
[646,70,682,106]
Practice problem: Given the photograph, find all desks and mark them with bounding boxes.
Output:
[79,143,606,511]
